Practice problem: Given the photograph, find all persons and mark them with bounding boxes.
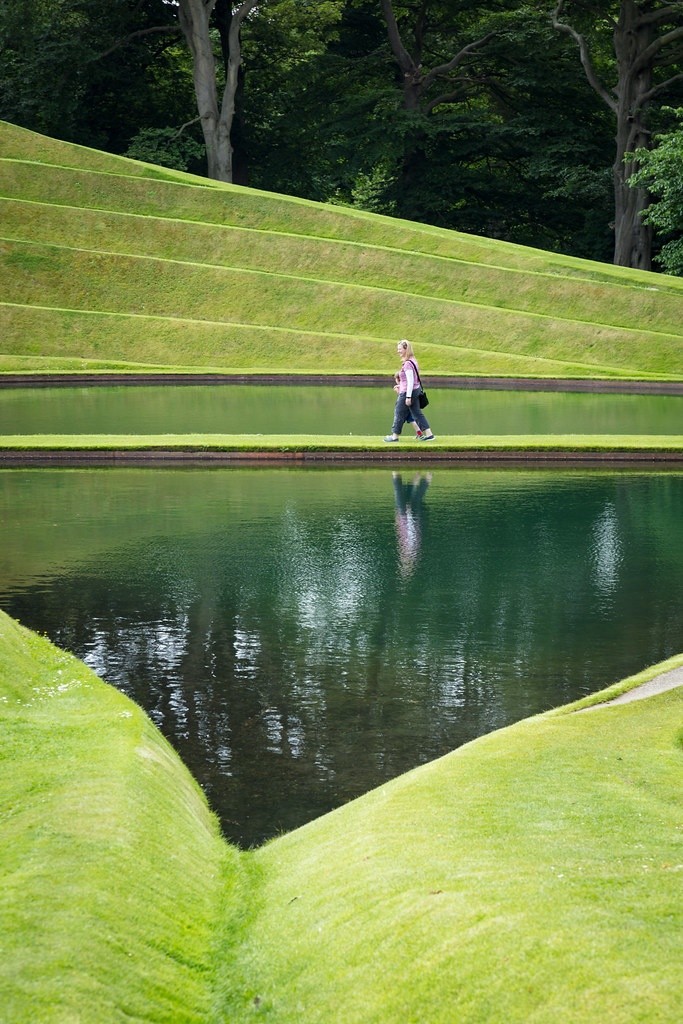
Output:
[391,470,432,592]
[384,340,435,442]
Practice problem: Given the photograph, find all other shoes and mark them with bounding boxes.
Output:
[414,434,423,439]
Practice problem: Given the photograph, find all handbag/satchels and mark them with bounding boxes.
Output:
[418,393,429,409]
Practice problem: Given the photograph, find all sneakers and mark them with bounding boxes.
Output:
[423,435,435,441]
[384,436,400,442]
